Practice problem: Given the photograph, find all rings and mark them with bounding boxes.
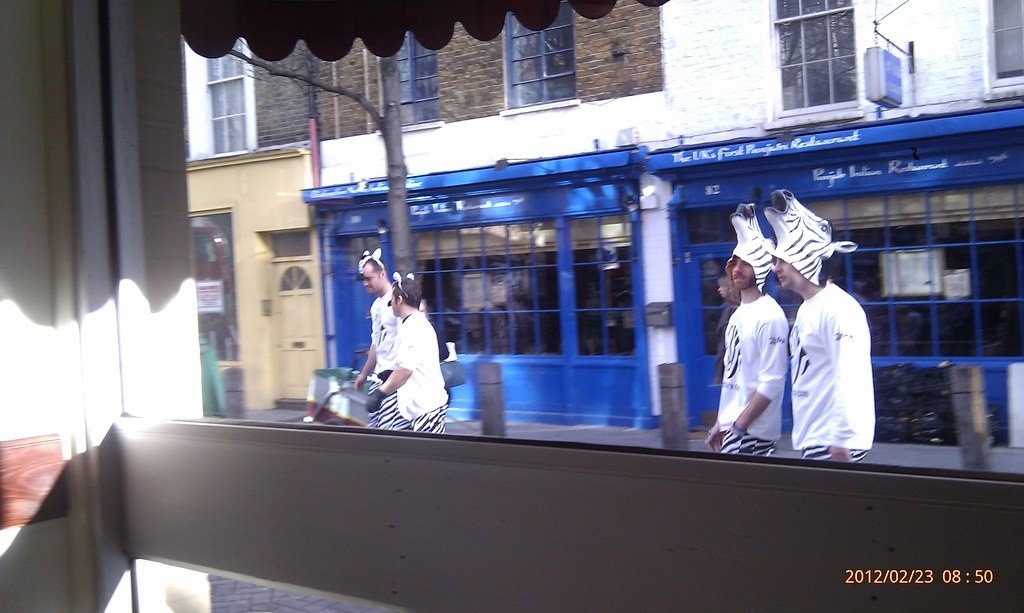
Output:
[708,430,711,435]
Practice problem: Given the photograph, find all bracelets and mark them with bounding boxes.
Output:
[733,420,748,435]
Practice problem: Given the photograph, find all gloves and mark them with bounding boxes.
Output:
[377,370,393,382]
[365,388,386,413]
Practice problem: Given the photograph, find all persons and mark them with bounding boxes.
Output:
[354,253,403,427]
[770,238,877,464]
[704,235,790,457]
[416,296,452,408]
[366,277,450,433]
[716,276,741,387]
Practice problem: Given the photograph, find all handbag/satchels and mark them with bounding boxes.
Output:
[439,360,466,388]
